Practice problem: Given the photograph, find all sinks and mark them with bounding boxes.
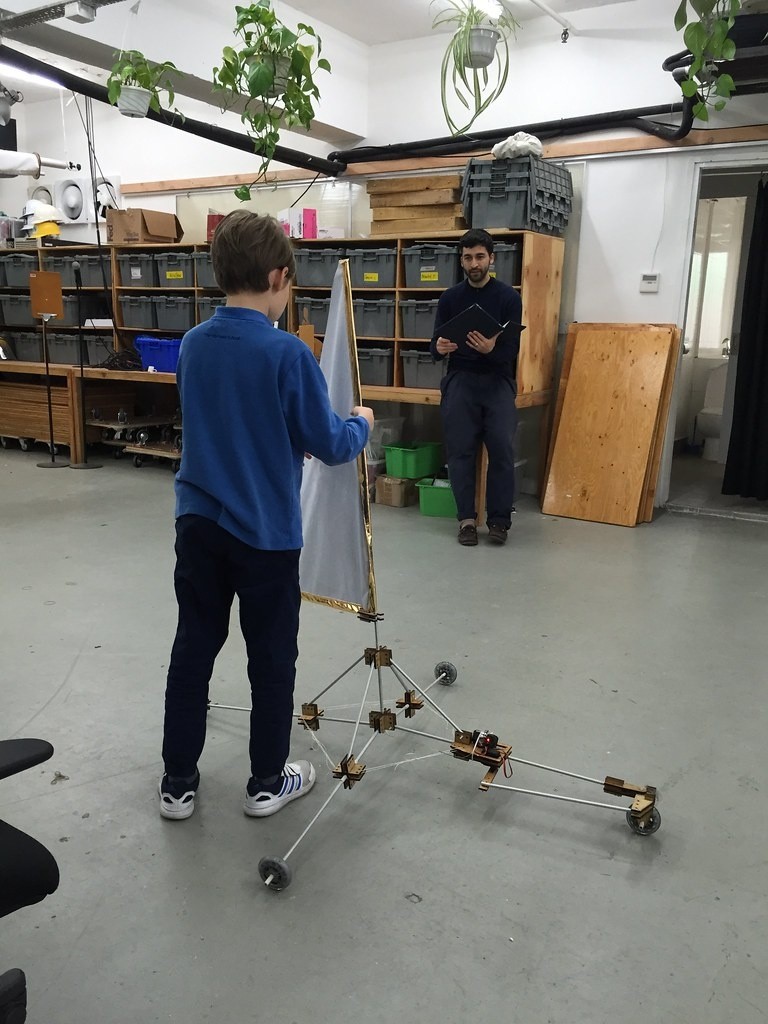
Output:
[693,357,729,370]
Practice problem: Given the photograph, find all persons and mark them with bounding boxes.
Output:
[430,229,522,545]
[158,208,374,819]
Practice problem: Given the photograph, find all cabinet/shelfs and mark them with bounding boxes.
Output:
[0,229,564,397]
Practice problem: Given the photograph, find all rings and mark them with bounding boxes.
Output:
[475,343,478,347]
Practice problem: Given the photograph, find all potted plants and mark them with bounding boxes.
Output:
[657,0,768,120]
[102,46,187,128]
[207,0,333,204]
[416,0,525,140]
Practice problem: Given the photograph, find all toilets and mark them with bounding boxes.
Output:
[697,363,728,461]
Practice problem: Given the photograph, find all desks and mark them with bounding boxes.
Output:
[0,360,90,462]
[74,368,557,525]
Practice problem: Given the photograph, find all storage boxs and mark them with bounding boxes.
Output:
[363,151,577,231]
[415,470,459,519]
[192,250,219,289]
[197,295,227,326]
[116,253,156,287]
[295,293,333,335]
[373,474,414,510]
[83,332,113,367]
[399,298,440,340]
[346,248,396,287]
[295,245,344,287]
[1,253,38,286]
[304,208,318,238]
[103,207,176,245]
[401,244,460,288]
[399,345,448,389]
[151,296,194,331]
[380,435,445,480]
[10,331,42,363]
[362,459,386,504]
[358,345,394,386]
[47,293,95,326]
[352,296,395,337]
[276,208,304,239]
[116,293,154,330]
[464,240,519,285]
[154,251,194,287]
[0,289,42,327]
[42,254,75,286]
[364,410,410,459]
[135,332,182,373]
[43,333,80,363]
[74,253,111,288]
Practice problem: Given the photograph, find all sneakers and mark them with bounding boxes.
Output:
[458,522,478,545]
[489,523,509,545]
[243,758,316,816]
[158,769,200,820]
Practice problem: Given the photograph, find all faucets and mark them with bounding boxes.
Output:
[721,338,730,358]
